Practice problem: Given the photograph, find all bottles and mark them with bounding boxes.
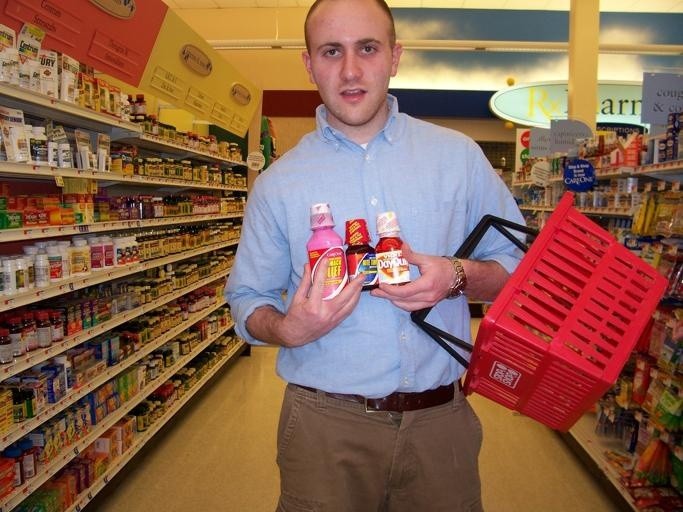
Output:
[25,257,35,285]
[23,312,39,351]
[88,237,107,270]
[134,93,146,114]
[36,312,52,348]
[3,260,15,294]
[34,241,47,254]
[127,95,136,115]
[341,218,378,292]
[57,244,71,279]
[6,316,26,357]
[1,329,13,364]
[73,239,92,276]
[113,235,141,266]
[35,254,50,288]
[375,212,412,289]
[102,237,115,270]
[46,246,63,280]
[16,258,29,291]
[304,201,349,303]
[57,240,71,248]
[47,240,57,247]
[51,312,65,342]
[23,245,37,254]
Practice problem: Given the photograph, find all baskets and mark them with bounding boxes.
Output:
[413,191,669,435]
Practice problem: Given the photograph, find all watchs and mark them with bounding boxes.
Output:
[442,253,467,299]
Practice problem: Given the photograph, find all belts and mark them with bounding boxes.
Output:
[286,381,463,411]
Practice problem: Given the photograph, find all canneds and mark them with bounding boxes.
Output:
[138,195,154,219]
[136,335,233,432]
[145,113,246,162]
[134,158,144,176]
[23,388,37,418]
[12,392,26,423]
[17,439,37,479]
[145,157,248,187]
[3,445,24,486]
[152,197,164,219]
[163,191,246,217]
[129,116,136,124]
[125,157,134,175]
[121,279,235,356]
[134,308,231,380]
[134,222,241,262]
[135,116,145,132]
[127,250,235,305]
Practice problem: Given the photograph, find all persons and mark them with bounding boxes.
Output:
[223,0,529,511]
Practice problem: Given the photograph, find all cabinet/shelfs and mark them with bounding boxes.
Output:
[510,161,683,512]
[0,81,251,512]
